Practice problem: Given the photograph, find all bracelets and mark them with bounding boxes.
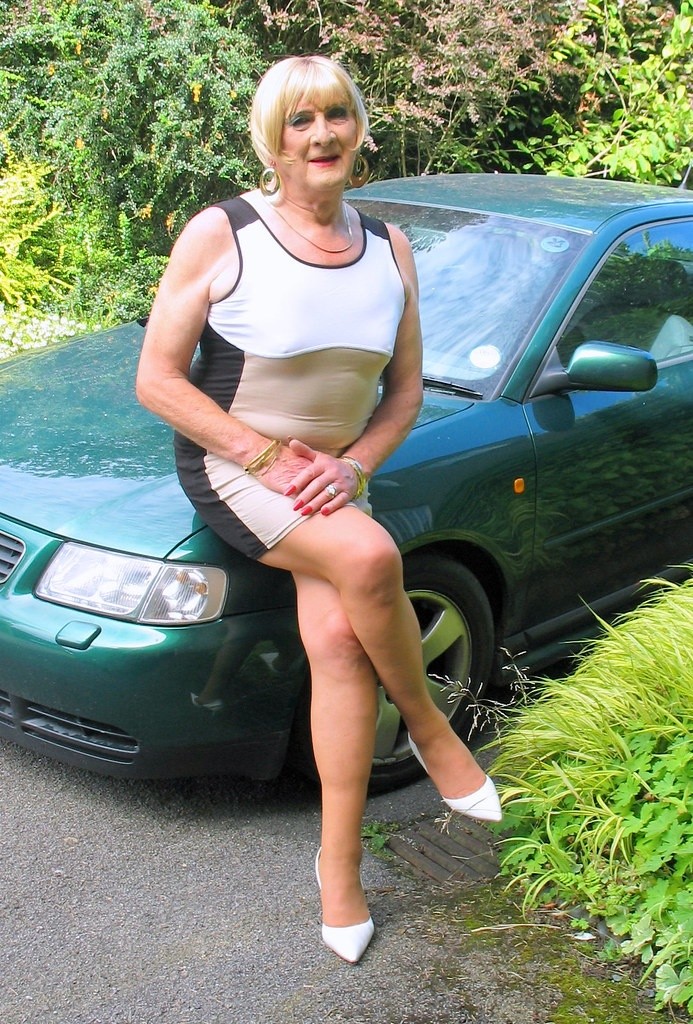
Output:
[339,455,370,501]
[244,439,282,477]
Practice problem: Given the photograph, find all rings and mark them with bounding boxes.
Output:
[326,485,336,499]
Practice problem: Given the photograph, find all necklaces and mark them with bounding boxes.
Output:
[260,193,354,253]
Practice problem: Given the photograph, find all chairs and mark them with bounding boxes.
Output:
[437,232,547,298]
[557,258,693,368]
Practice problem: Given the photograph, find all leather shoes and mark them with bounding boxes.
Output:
[406,732,504,822]
[315,846,375,965]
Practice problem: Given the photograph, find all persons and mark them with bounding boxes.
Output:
[134,56,503,962]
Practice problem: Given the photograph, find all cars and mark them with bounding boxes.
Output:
[0,172,693,799]
